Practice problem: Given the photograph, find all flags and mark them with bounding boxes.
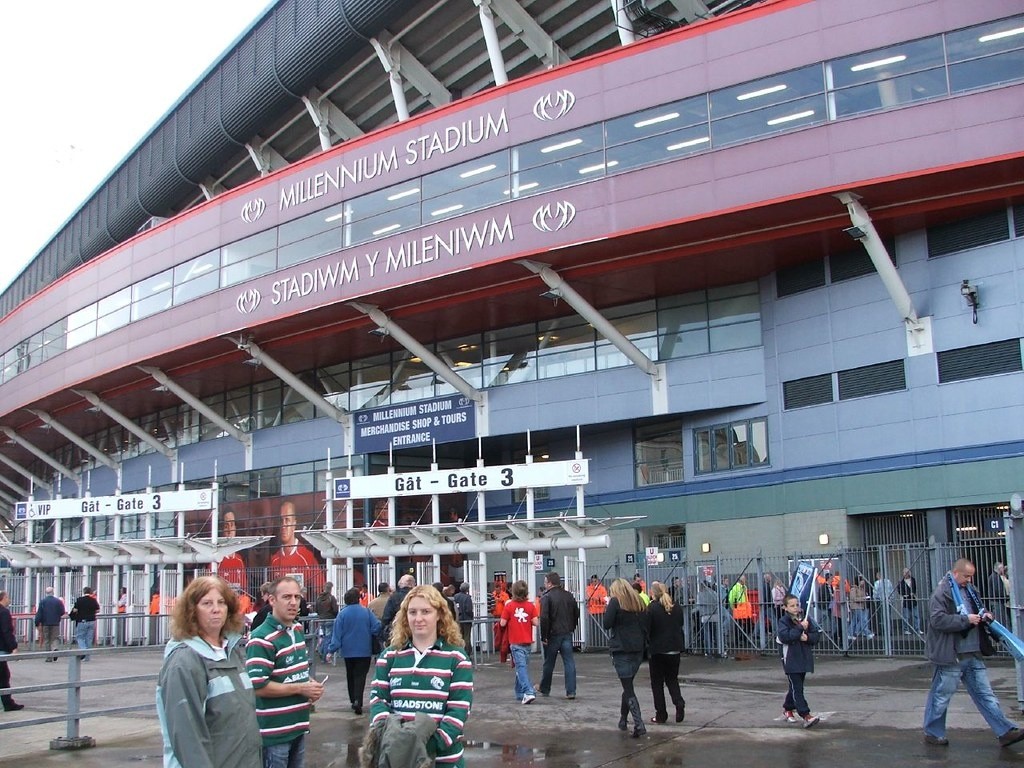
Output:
[788,561,815,609]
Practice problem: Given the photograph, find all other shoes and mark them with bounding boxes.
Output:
[848,636,857,640]
[650,716,667,723]
[565,694,575,699]
[675,705,684,722]
[782,710,798,723]
[924,735,949,745]
[867,633,875,640]
[45,649,59,662]
[521,693,535,705]
[352,700,363,715]
[80,655,85,660]
[533,683,549,696]
[998,727,1024,748]
[4,703,24,712]
[803,716,820,728]
[905,631,911,635]
[916,631,924,634]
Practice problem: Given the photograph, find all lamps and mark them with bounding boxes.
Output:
[7,439,17,444]
[242,359,262,366]
[369,328,389,337]
[153,386,168,392]
[539,291,563,299]
[38,424,51,429]
[86,406,100,413]
[844,226,867,241]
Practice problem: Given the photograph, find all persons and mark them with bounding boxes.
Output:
[250,582,273,632]
[923,558,1024,748]
[119,587,127,613]
[73,587,100,661]
[369,585,474,768]
[646,581,685,723]
[372,500,388,564]
[155,576,263,768]
[603,577,646,738]
[270,502,325,593]
[150,588,160,614]
[487,573,684,704]
[446,506,468,568]
[207,506,246,596]
[316,574,474,715]
[687,562,1012,728]
[245,577,324,768]
[35,587,65,662]
[0,591,24,712]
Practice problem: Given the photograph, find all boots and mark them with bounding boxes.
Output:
[626,696,647,737]
[618,692,629,731]
